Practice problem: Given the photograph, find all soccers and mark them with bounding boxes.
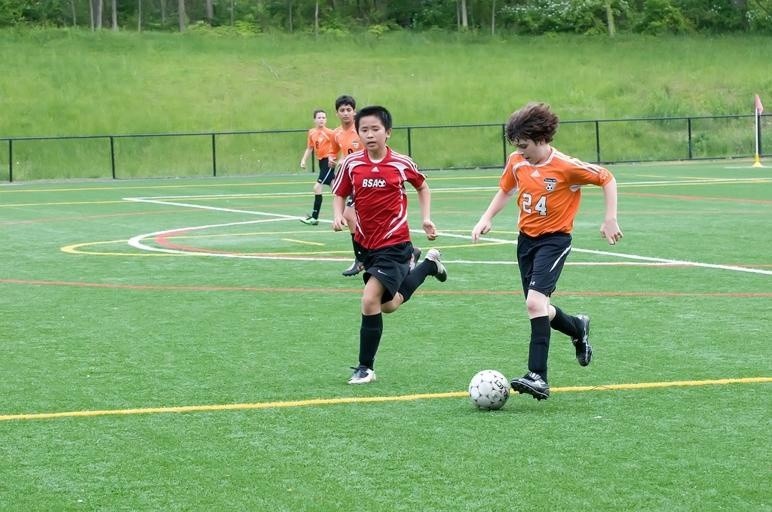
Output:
[469,370,510,409]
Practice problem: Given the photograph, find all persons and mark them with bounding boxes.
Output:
[299,110,336,226]
[471,102,623,401]
[327,94,366,277]
[330,107,448,386]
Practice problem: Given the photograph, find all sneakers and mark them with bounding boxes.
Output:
[300,215,319,225]
[342,258,364,277]
[572,315,592,366]
[409,247,447,282]
[346,365,376,384]
[345,196,353,206]
[511,371,549,401]
